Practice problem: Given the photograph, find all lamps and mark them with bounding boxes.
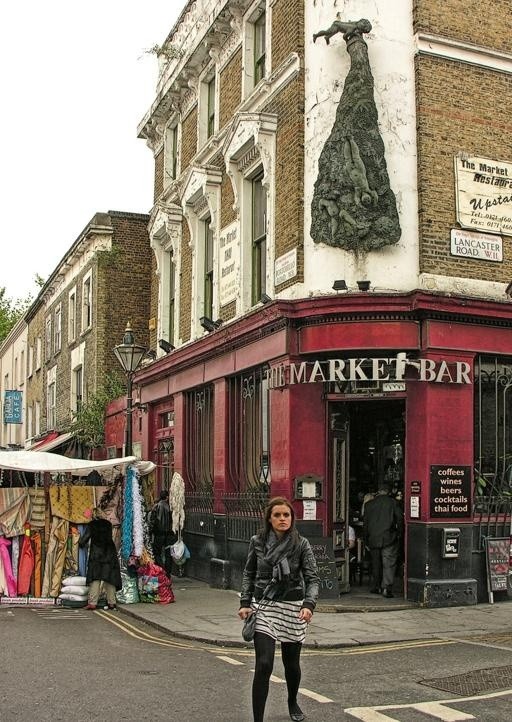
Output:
[332,278,371,293]
[199,316,219,332]
[158,339,175,353]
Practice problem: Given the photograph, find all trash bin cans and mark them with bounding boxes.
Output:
[486,537,511,591]
[429,465,471,518]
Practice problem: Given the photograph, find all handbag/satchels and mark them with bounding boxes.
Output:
[242,611,257,642]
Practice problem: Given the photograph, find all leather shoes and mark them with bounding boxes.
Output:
[370,588,394,598]
[83,604,96,610]
[108,603,116,609]
[288,700,304,722]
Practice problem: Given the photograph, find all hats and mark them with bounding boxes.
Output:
[170,540,191,566]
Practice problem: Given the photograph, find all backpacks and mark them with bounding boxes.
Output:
[153,504,163,537]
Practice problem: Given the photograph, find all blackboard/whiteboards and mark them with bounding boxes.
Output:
[305,536,339,598]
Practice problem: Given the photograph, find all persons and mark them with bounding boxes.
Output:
[150,491,177,587]
[77,508,123,611]
[237,496,319,722]
[313,19,372,45]
[348,484,404,598]
[146,498,159,561]
